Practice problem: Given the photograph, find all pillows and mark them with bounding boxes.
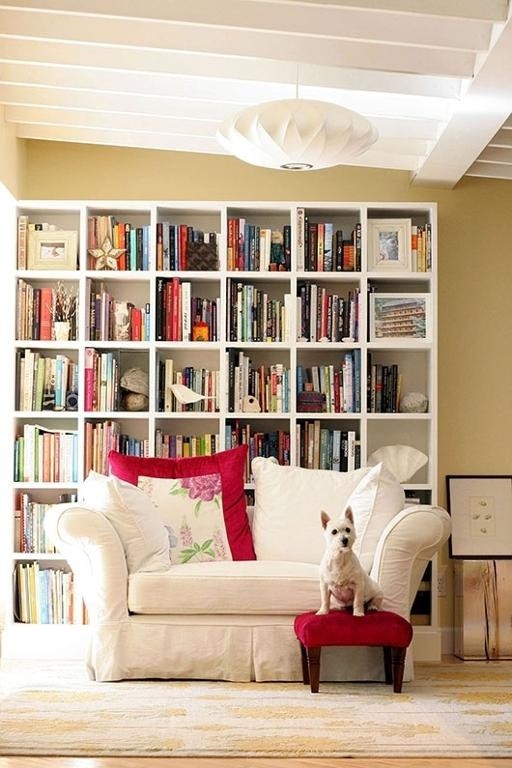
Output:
[107,444,256,560]
[250,457,383,560]
[84,472,171,568]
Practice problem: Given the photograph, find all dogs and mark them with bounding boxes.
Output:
[315,504,385,616]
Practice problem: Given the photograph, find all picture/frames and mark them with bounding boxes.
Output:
[446,475,512,558]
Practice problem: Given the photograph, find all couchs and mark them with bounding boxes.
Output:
[45,445,449,683]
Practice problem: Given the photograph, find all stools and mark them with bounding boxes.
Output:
[294,606,413,693]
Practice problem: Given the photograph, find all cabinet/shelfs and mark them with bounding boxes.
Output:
[9,201,442,663]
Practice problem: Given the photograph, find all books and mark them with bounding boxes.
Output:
[88,216,149,271]
[404,489,432,508]
[18,216,77,270]
[228,218,291,271]
[157,222,221,271]
[367,353,401,413]
[367,280,430,342]
[244,489,254,506]
[16,420,148,483]
[367,218,431,276]
[157,277,220,342]
[409,574,432,625]
[296,282,359,342]
[15,277,78,413]
[296,348,360,472]
[225,349,290,466]
[228,277,289,341]
[15,491,78,552]
[154,350,220,458]
[297,210,360,273]
[12,561,88,625]
[84,278,150,412]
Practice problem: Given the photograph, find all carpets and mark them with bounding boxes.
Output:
[1,656,512,757]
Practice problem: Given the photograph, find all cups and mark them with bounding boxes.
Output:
[54,322,70,342]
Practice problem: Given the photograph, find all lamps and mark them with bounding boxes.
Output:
[215,64,379,171]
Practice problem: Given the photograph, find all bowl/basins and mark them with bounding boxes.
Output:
[126,393,146,411]
